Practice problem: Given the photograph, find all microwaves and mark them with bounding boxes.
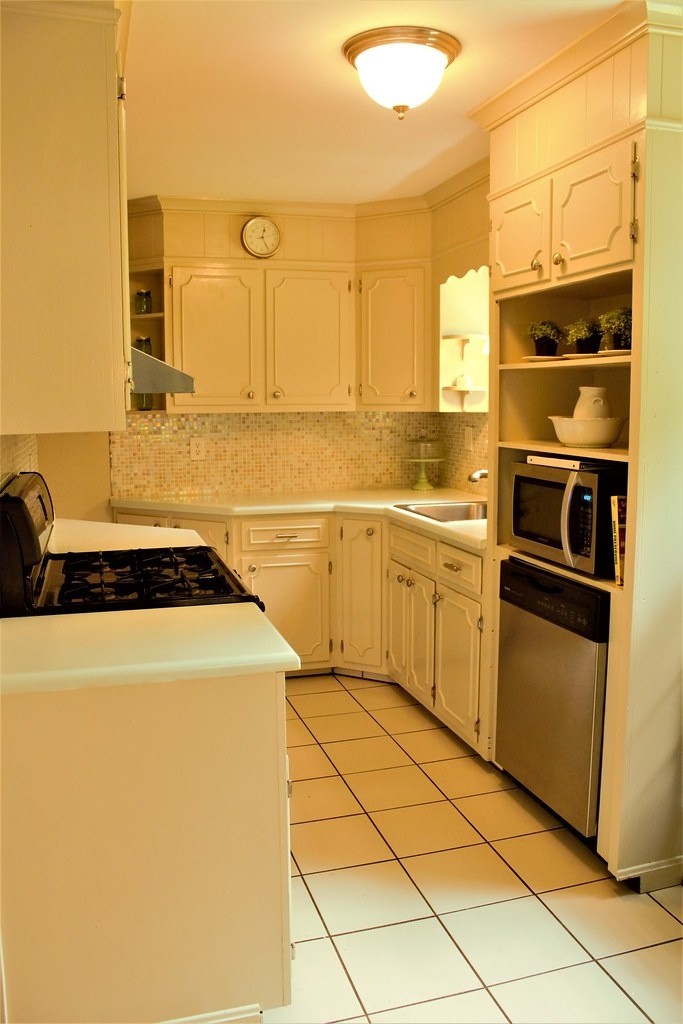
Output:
[509,461,627,577]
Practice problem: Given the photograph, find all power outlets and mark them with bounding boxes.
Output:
[189,437,207,462]
[466,426,474,449]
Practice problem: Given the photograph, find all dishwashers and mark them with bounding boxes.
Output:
[493,558,612,840]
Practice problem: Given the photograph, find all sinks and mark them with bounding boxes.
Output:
[392,501,488,523]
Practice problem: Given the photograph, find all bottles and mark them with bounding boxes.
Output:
[136,336,153,357]
[135,287,153,313]
[574,385,612,417]
[138,392,153,411]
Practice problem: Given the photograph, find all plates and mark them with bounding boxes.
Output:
[521,349,630,362]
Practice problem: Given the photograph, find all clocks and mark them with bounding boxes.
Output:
[241,217,282,258]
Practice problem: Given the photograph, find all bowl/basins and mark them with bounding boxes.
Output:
[549,415,629,448]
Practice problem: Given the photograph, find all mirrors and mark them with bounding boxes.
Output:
[436,265,492,411]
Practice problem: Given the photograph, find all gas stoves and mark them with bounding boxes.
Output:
[0,469,265,619]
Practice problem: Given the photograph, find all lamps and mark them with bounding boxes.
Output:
[340,27,462,120]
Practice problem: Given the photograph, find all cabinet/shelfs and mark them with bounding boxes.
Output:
[434,542,483,744]
[0,671,294,1024]
[355,267,426,413]
[484,139,638,291]
[127,264,164,414]
[239,520,333,665]
[402,429,447,493]
[386,525,439,713]
[489,269,647,590]
[342,517,384,668]
[0,0,136,433]
[113,512,227,568]
[169,266,355,412]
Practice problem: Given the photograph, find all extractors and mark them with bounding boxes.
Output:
[130,345,197,394]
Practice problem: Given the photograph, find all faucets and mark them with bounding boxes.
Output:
[468,469,489,483]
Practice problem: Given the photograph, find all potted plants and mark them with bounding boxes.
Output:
[566,321,603,354]
[523,319,566,354]
[600,307,634,358]
